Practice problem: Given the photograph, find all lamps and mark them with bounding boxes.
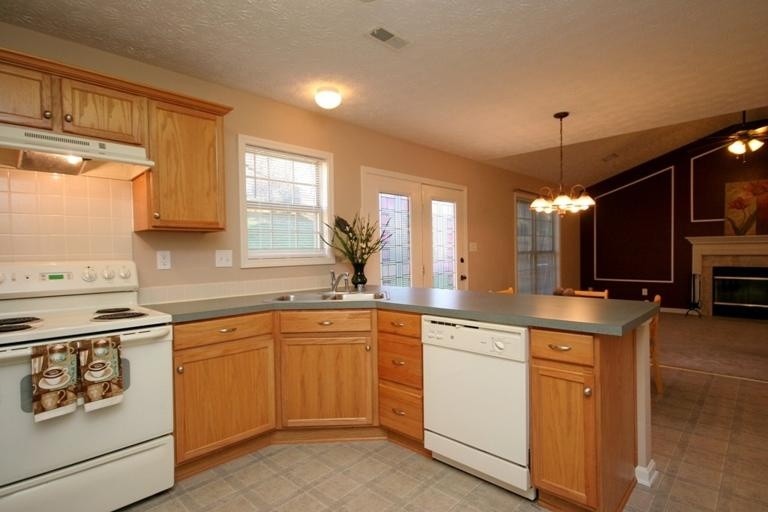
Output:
[314,88,342,112]
[529,111,595,219]
[725,139,765,165]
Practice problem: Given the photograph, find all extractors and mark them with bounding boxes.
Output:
[1,121,156,182]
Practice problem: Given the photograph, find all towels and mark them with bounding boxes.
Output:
[30,341,80,425]
[78,334,126,411]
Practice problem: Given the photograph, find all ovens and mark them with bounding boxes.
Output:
[0,324,175,511]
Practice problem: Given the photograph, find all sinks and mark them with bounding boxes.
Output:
[261,291,331,302]
[331,290,391,302]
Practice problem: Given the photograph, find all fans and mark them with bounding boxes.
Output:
[699,110,768,149]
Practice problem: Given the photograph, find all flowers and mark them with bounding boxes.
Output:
[319,213,396,263]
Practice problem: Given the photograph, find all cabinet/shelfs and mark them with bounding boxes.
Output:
[174,305,276,479]
[378,309,430,458]
[529,325,637,512]
[275,305,378,444]
[134,83,233,232]
[0,47,142,144]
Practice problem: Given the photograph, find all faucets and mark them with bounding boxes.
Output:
[329,269,349,291]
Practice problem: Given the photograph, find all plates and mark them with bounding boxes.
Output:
[84,366,114,383]
[37,374,71,391]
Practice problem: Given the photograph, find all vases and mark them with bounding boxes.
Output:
[350,262,367,288]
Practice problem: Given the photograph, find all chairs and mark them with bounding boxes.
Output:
[648,292,666,393]
[554,287,608,299]
[488,286,514,294]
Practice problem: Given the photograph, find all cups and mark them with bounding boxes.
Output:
[86,382,111,401]
[31,354,44,375]
[40,389,67,412]
[87,360,112,378]
[47,343,73,366]
[91,337,117,362]
[78,347,89,367]
[42,365,69,385]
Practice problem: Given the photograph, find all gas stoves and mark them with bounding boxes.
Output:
[0,304,172,344]
